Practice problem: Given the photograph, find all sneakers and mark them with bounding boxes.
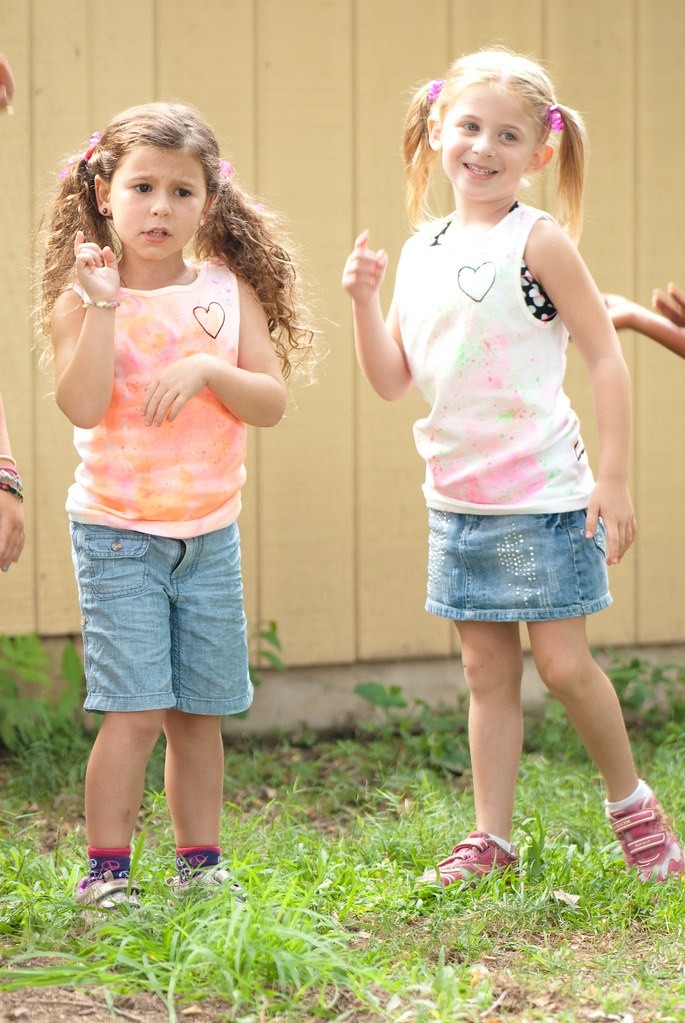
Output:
[604,788,685,884]
[165,866,243,893]
[417,830,519,889]
[75,869,141,911]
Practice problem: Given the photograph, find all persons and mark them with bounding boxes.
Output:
[0,58,24,572]
[601,281,685,359]
[36,102,317,908]
[343,52,685,892]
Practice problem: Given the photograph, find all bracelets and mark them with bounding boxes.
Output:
[0,455,24,503]
[87,299,120,308]
